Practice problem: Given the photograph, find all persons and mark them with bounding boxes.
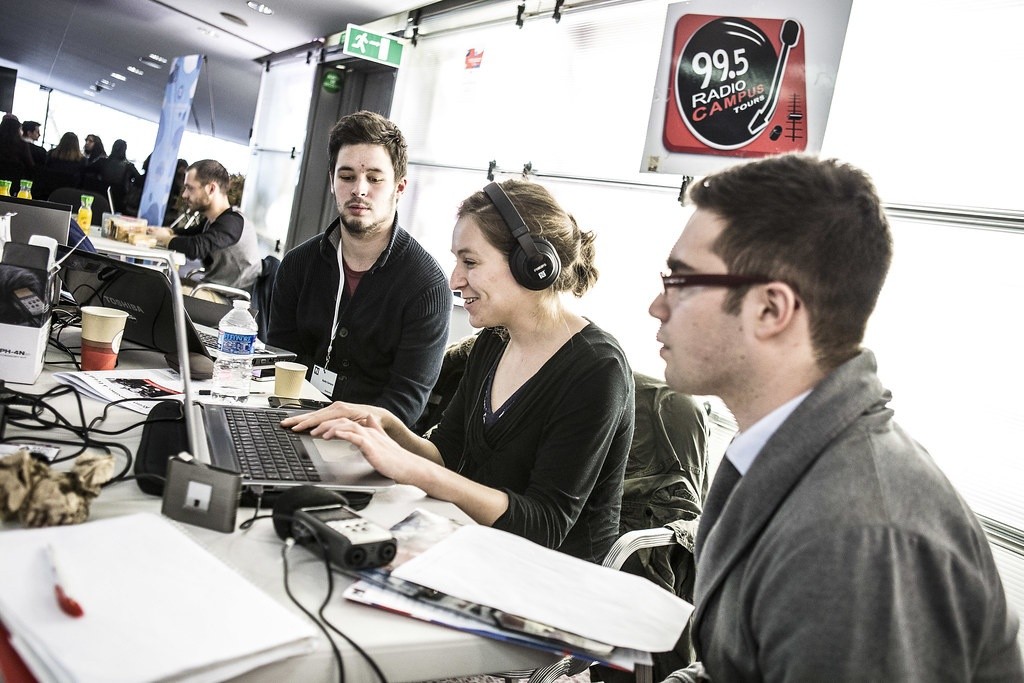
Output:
[266,111,454,428]
[146,160,262,326]
[649,157,1024,683]
[0,114,188,202]
[281,180,635,565]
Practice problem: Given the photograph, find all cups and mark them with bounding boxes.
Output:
[275,362,308,400]
[101,211,122,237]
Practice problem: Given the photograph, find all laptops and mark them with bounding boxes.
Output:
[168,254,396,495]
[55,243,298,365]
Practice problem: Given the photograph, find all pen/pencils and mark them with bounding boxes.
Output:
[198,387,266,398]
[47,543,84,619]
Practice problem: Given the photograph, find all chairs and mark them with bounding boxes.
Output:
[485,369,710,683]
[169,235,280,345]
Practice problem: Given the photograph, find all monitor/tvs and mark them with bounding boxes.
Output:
[107,186,115,214]
[0,195,73,306]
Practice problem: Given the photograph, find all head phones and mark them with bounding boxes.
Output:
[484,182,561,292]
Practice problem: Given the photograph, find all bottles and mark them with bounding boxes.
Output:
[77,196,95,236]
[211,300,259,408]
[81,306,129,371]
[17,180,33,200]
[0,180,12,197]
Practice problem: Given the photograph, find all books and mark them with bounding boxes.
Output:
[51,367,212,415]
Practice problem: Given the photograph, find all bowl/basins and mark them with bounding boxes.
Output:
[128,233,157,248]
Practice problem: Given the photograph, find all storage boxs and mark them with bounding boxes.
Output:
[0,231,58,384]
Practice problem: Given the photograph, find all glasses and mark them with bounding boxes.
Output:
[660,272,801,310]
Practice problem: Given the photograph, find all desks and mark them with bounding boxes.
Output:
[0,329,555,683]
[87,221,185,282]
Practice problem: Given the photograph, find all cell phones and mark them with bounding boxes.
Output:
[242,367,276,381]
[268,397,331,410]
